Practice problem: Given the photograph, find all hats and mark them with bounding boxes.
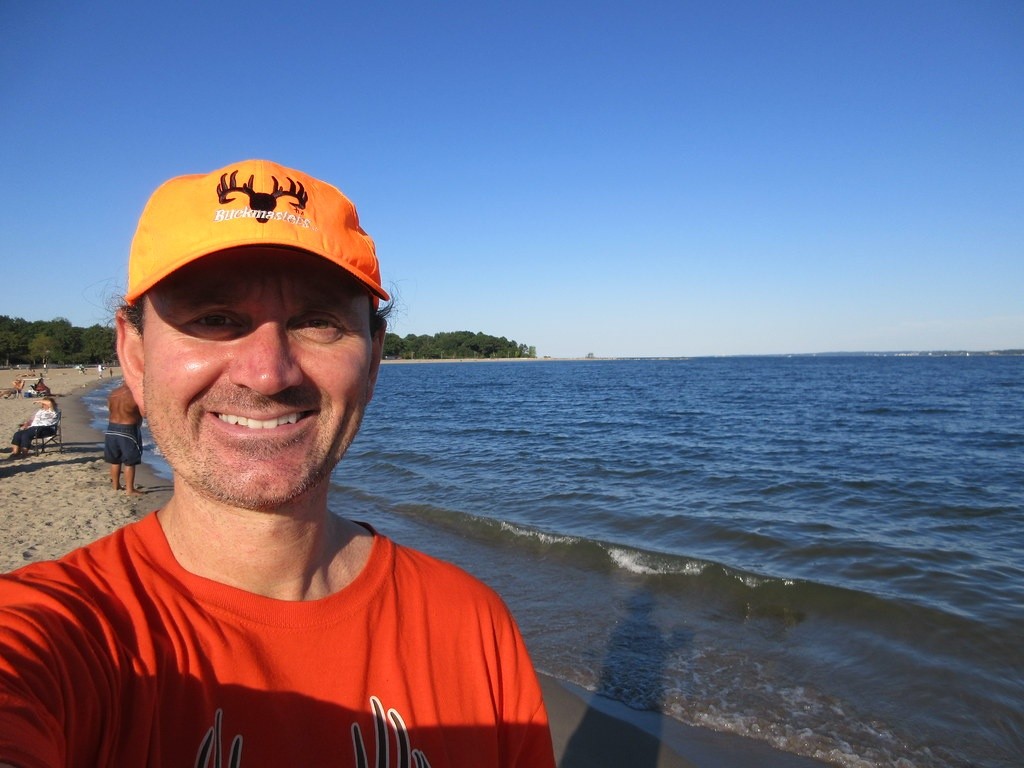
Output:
[123,160,390,316]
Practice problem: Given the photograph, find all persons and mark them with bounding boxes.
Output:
[0,159,557,768]
[0,362,114,398]
[7,397,61,461]
[102,380,150,496]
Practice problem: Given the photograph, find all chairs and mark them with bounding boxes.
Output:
[31,384,45,397]
[32,412,64,457]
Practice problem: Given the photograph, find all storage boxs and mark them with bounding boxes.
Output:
[23,392,32,398]
